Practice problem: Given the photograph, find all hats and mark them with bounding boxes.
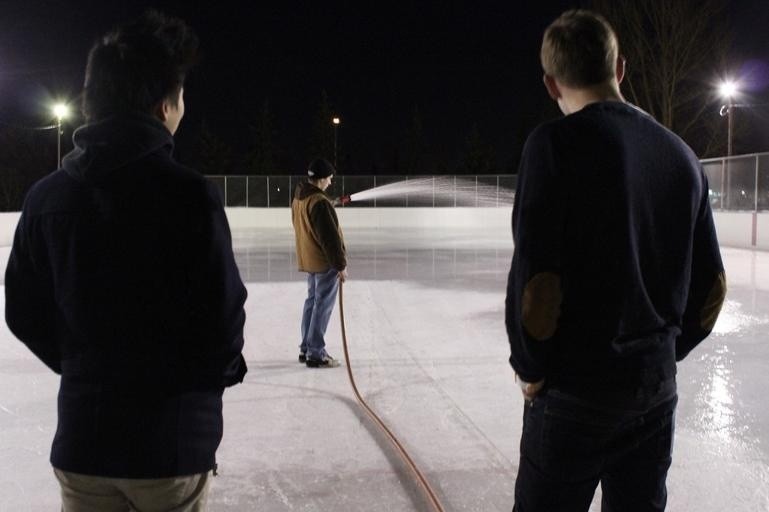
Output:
[307,156,337,180]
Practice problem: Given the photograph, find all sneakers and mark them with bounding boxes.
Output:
[299,354,341,370]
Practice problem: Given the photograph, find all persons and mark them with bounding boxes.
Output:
[504,7,728,512]
[291,156,349,368]
[3,22,250,512]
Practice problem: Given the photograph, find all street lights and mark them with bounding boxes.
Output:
[53,103,68,168]
[82,28,120,114]
[718,80,739,210]
[331,114,340,173]
